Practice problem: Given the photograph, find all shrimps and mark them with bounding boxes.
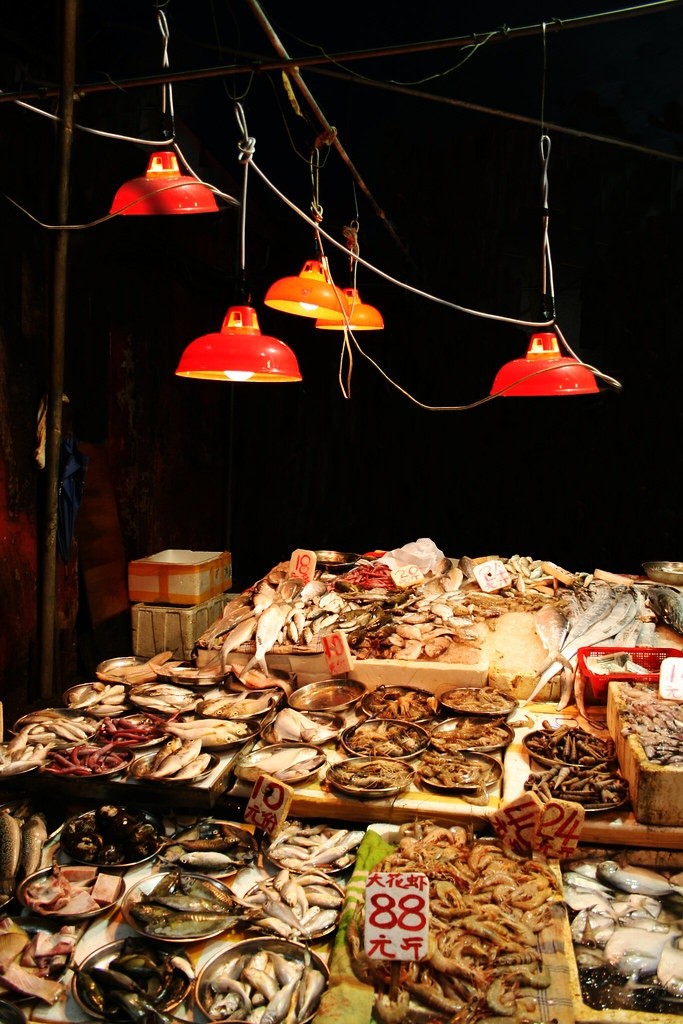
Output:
[338,685,535,807]
[344,819,553,1024]
[617,682,683,767]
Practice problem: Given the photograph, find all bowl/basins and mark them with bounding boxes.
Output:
[642,561,683,585]
[311,550,362,572]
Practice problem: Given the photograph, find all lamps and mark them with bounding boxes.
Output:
[176,305,305,385]
[109,152,221,216]
[265,258,354,321]
[316,287,383,336]
[491,333,601,396]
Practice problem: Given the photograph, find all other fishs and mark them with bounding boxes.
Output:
[560,856,683,1015]
[71,821,365,1024]
[25,865,124,916]
[0,798,48,897]
[233,708,338,783]
[0,650,277,785]
[203,555,552,675]
[524,577,683,729]
[0,917,77,1006]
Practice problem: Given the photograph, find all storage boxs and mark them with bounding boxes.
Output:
[134,594,228,655]
[348,649,490,691]
[129,549,232,605]
[609,681,683,828]
[195,648,334,688]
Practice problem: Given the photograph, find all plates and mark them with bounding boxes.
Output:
[0,658,631,815]
[0,811,360,1023]
